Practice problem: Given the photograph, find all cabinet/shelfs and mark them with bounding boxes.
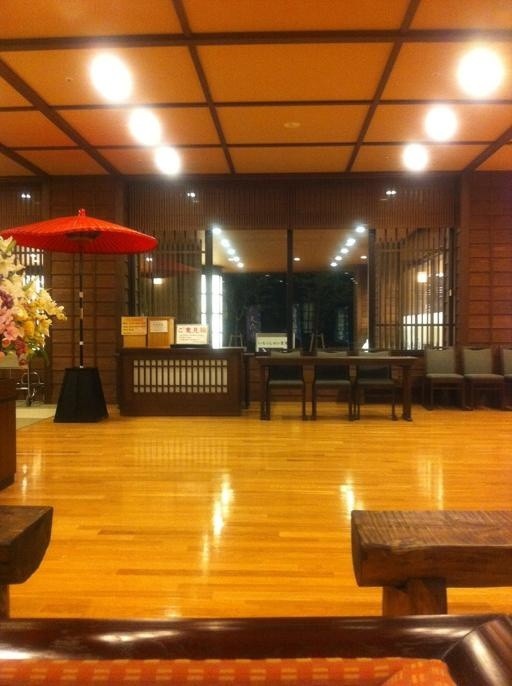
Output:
[0,377,19,491]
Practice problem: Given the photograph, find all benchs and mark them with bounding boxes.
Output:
[351,510,511,614]
[0,503,54,616]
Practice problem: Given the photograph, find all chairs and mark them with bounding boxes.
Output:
[467,344,501,411]
[423,344,467,412]
[312,351,353,421]
[501,344,511,411]
[265,348,308,421]
[355,350,403,421]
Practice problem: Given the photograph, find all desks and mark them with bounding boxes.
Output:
[252,348,417,422]
[1,614,511,685]
[116,347,248,417]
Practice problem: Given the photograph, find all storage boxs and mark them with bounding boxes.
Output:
[121,316,146,348]
[147,316,176,349]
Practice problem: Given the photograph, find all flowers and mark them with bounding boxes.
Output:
[0,235,68,359]
